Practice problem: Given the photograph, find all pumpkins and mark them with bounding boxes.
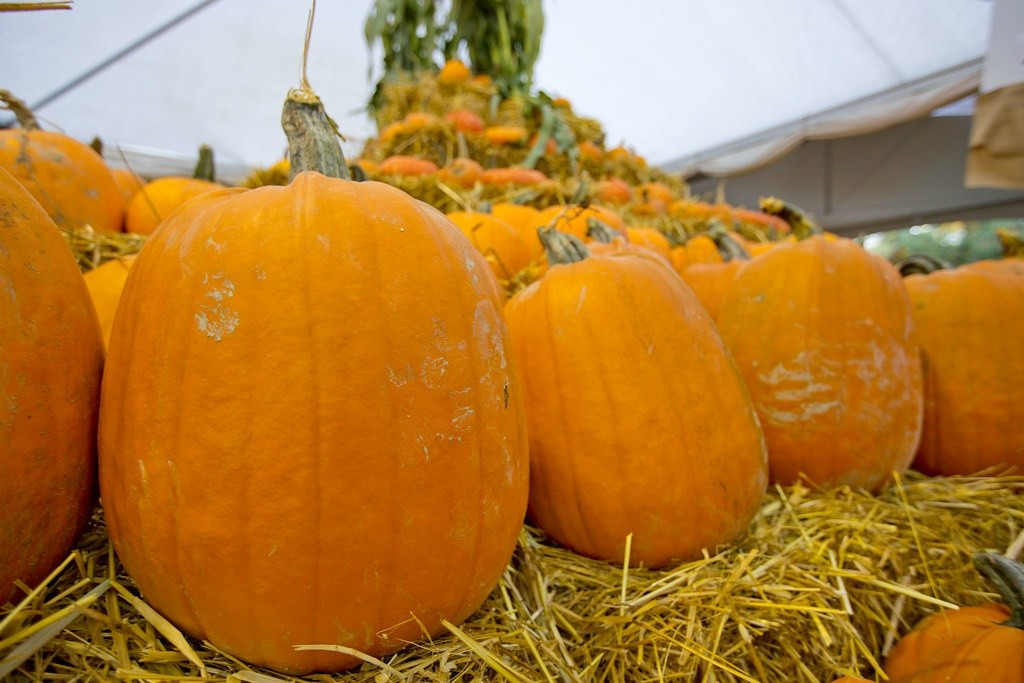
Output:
[0,58,1024,683]
